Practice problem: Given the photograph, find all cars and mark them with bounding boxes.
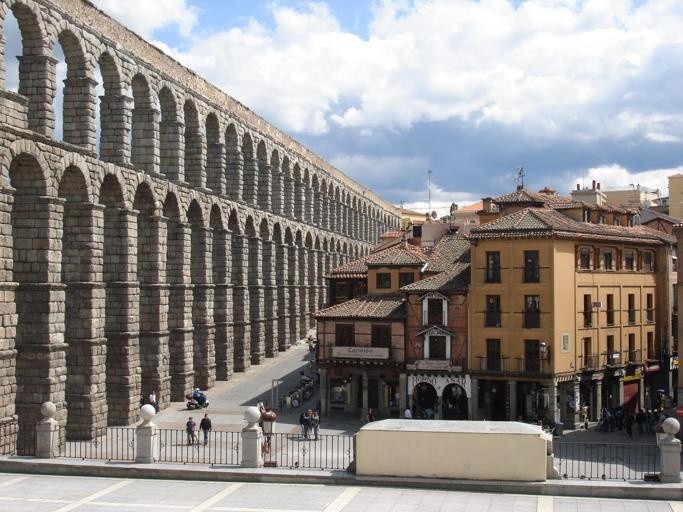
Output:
[305,329,317,343]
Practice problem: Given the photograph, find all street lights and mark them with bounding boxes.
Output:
[671,351,679,406]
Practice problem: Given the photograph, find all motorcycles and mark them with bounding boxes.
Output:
[184,392,209,410]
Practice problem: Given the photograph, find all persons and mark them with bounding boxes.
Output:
[140,393,144,405]
[184,416,197,446]
[403,405,411,419]
[600,406,658,439]
[198,414,211,445]
[366,407,374,423]
[148,390,157,406]
[299,409,319,441]
[257,400,265,414]
[193,388,201,401]
